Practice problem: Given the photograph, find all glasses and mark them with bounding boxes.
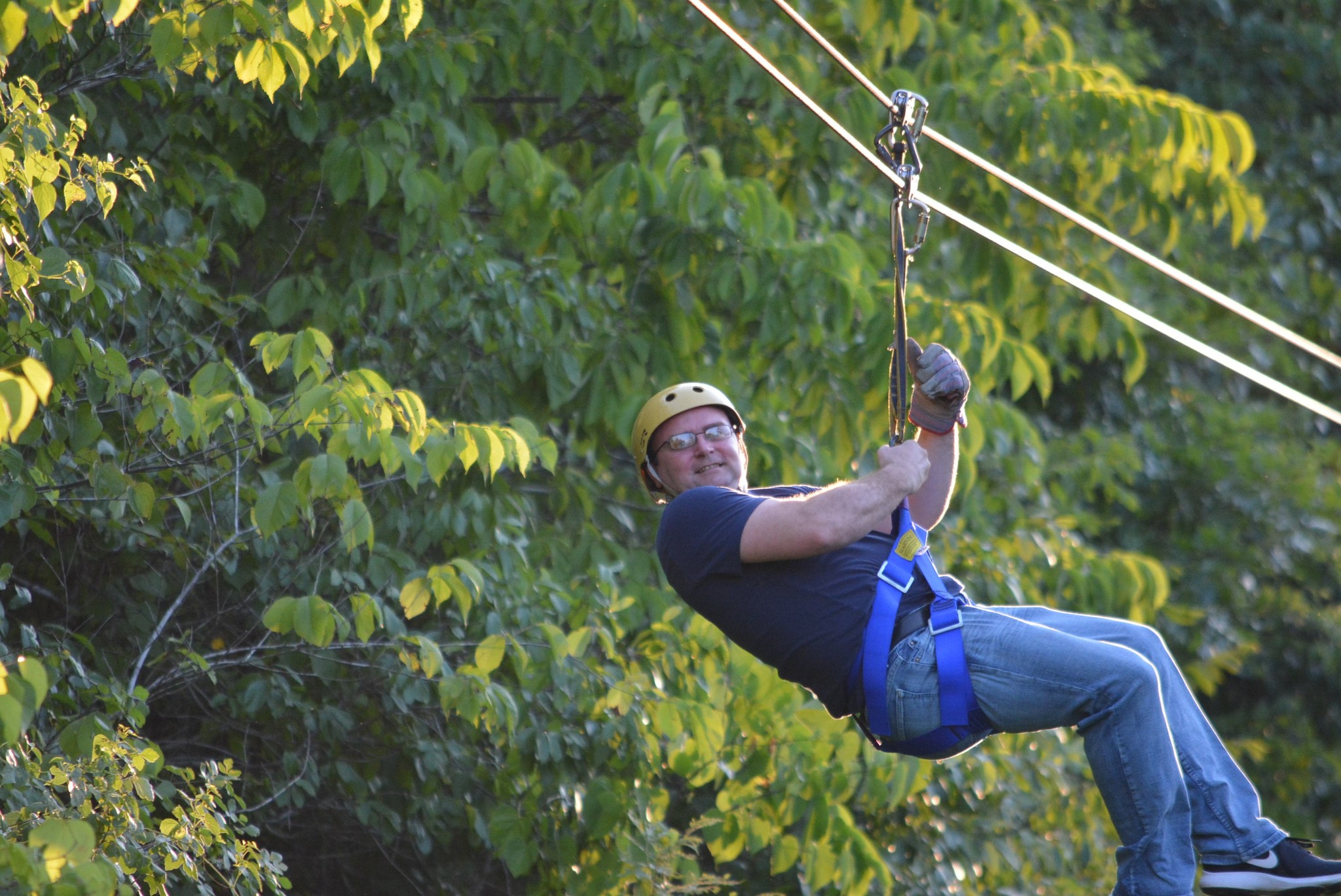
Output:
[652,425,738,457]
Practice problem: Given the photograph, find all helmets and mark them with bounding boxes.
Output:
[631,382,747,493]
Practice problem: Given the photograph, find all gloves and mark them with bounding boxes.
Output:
[905,337,970,433]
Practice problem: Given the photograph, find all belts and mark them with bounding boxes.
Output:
[890,603,931,650]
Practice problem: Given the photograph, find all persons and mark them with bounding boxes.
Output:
[633,335,1341,896]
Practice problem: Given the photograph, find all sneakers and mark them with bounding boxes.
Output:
[1199,838,1341,896]
